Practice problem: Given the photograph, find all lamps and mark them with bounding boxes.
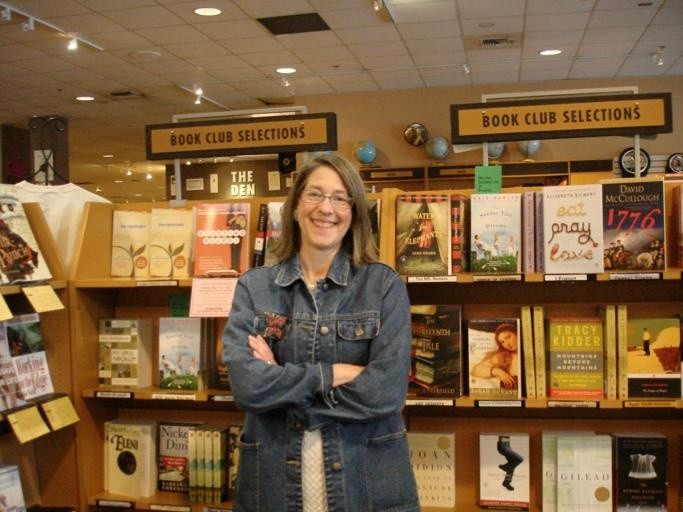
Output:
[0,1,81,54]
[193,83,203,108]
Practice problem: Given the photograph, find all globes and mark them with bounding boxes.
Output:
[352,141,377,168]
[488,141,505,164]
[425,136,449,166]
[517,140,540,162]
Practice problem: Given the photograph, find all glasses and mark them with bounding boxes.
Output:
[299,189,353,209]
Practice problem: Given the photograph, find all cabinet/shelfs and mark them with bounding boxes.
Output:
[0,179,71,512]
[72,185,390,511]
[384,174,682,512]
[355,156,616,191]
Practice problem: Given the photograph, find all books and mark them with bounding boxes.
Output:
[104,418,244,504]
[364,178,683,278]
[409,431,456,508]
[480,432,530,508]
[0,313,55,411]
[97,291,231,394]
[111,201,284,278]
[408,303,682,399]
[0,464,27,512]
[0,193,53,287]
[543,430,612,511]
[610,432,667,511]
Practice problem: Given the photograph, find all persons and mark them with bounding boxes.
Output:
[497,435,524,490]
[222,151,421,512]
